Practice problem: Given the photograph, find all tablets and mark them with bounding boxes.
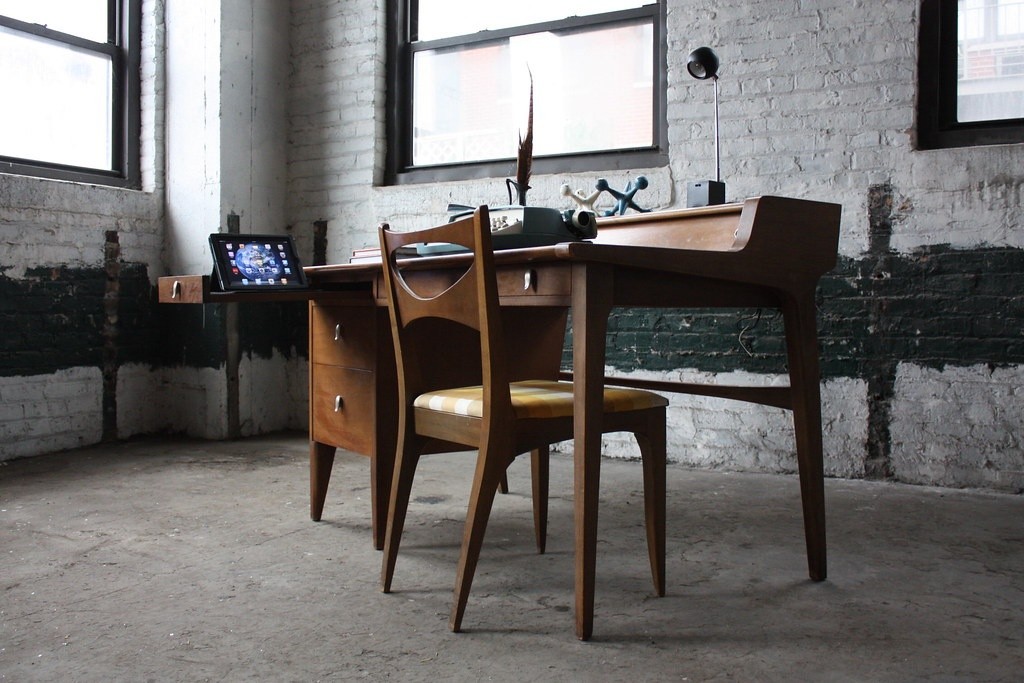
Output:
[209,233,310,291]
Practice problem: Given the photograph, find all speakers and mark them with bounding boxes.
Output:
[687,180,725,209]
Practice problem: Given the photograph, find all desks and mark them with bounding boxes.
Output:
[158,195,847,582]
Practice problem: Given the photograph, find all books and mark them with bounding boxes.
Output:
[348,245,420,265]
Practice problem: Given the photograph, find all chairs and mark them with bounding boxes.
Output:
[372,204,671,634]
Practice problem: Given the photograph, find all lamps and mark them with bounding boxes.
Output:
[687,47,726,208]
